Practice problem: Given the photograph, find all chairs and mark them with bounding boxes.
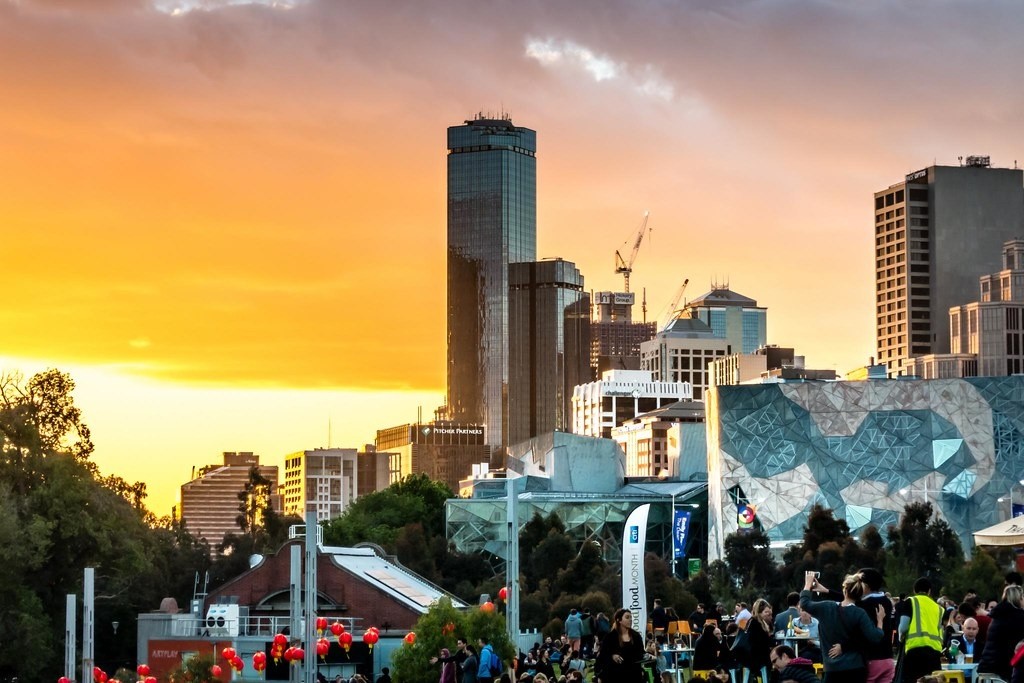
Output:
[655,619,718,649]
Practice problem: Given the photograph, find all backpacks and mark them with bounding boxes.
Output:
[484,647,503,677]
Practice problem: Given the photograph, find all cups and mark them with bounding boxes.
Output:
[534,628,537,634]
[676,644,682,650]
[966,654,973,664]
[958,654,964,665]
[526,629,529,634]
[519,629,521,634]
[663,643,668,651]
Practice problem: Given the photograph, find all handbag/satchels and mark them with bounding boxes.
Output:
[729,618,756,665]
[593,650,615,681]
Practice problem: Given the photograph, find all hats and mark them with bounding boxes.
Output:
[859,568,883,586]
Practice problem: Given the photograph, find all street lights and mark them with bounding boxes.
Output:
[672,503,701,576]
[999,497,1013,518]
[737,499,757,530]
[901,489,954,506]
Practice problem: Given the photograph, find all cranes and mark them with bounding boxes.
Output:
[658,279,690,333]
[614,211,649,294]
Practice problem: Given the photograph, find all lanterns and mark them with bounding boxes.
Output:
[58,586,507,683]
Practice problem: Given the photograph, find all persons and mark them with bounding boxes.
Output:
[317,568,1024,683]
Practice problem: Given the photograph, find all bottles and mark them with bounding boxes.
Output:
[949,639,960,663]
[786,615,794,637]
[804,624,810,637]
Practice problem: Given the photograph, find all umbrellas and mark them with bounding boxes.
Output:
[972,513,1024,548]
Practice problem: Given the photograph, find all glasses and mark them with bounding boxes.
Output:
[698,608,700,610]
[772,656,779,664]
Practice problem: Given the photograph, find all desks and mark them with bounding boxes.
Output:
[774,635,813,656]
[942,663,979,683]
[659,649,695,683]
[723,618,735,625]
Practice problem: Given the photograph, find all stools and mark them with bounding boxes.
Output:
[644,668,655,683]
[664,668,684,683]
[977,671,999,683]
[693,671,712,679]
[743,666,767,683]
[932,669,967,683]
